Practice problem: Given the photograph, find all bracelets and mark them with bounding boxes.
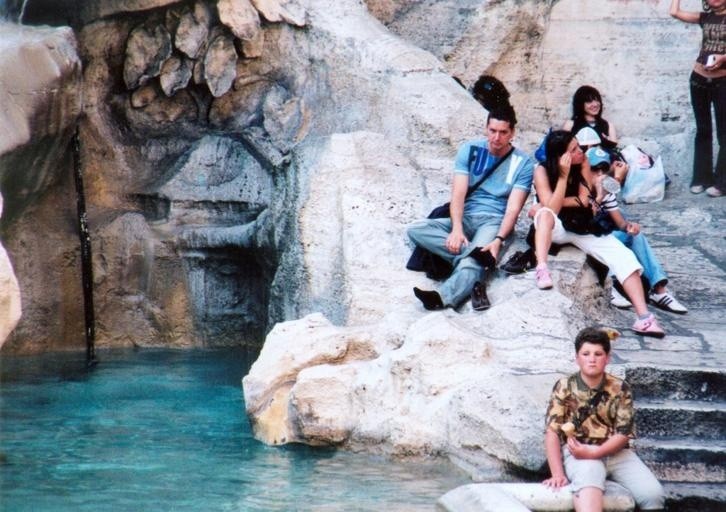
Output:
[495,236,504,241]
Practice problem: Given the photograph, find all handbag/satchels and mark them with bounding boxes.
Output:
[406,204,450,283]
[622,166,671,191]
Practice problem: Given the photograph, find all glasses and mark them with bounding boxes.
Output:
[590,165,610,172]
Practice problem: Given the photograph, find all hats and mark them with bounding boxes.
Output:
[585,145,612,168]
[576,126,601,147]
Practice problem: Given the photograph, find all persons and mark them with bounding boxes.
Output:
[406,106,534,310]
[669,0,726,196]
[530,85,688,336]
[542,329,664,512]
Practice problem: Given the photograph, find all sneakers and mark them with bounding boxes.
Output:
[690,184,703,194]
[534,264,552,290]
[499,248,537,273]
[630,311,663,338]
[649,290,688,315]
[609,284,632,308]
[469,279,489,311]
[706,187,722,197]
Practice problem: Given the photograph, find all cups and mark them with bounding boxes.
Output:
[601,176,621,194]
